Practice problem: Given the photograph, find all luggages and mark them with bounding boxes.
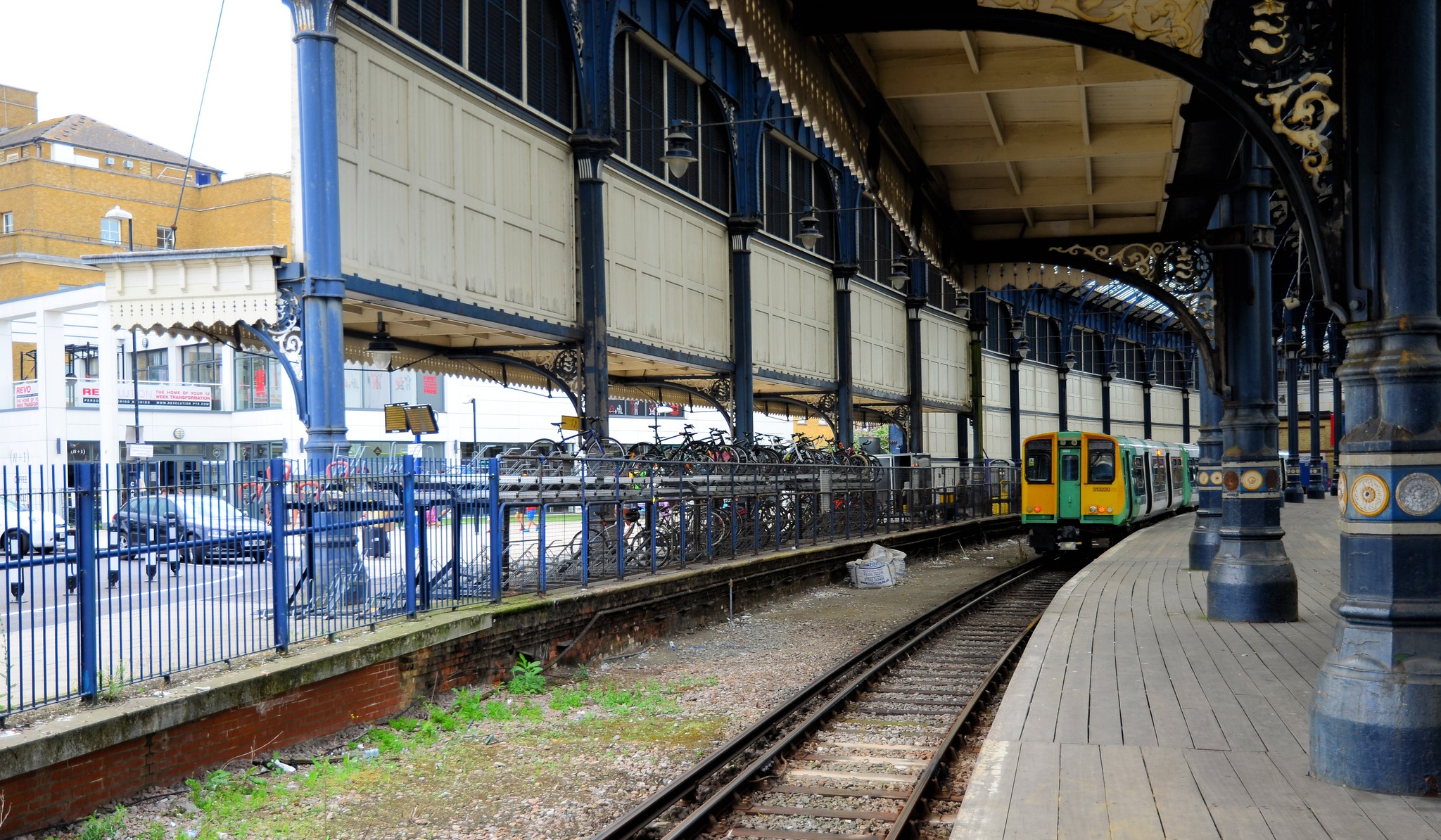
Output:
[363,527,385,558]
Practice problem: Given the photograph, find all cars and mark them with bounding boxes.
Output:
[107,494,273,566]
[1,496,64,560]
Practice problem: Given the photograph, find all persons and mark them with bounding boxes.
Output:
[946,478,969,520]
[178,489,185,494]
[262,502,272,565]
[438,507,451,521]
[621,502,639,546]
[523,506,539,532]
[161,488,170,495]
[486,516,490,533]
[515,507,526,531]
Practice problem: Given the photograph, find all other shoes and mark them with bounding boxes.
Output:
[428,522,435,526]
[263,558,272,565]
[519,527,524,531]
[487,530,490,532]
[536,527,538,531]
[524,529,529,532]
[386,553,390,558]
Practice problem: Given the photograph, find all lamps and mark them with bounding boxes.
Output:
[173,428,185,438]
[1185,377,1197,393]
[282,438,288,453]
[1065,350,1077,370]
[795,207,824,248]
[57,438,61,454]
[887,259,911,289]
[951,296,971,318]
[660,118,700,178]
[142,338,148,348]
[1016,340,1030,357]
[1147,371,1158,386]
[1009,317,1024,339]
[454,440,458,454]
[1107,361,1119,378]
[300,438,304,452]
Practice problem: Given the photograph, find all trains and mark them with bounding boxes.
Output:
[1019,424,1338,559]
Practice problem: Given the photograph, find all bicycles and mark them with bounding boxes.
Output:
[492,418,887,564]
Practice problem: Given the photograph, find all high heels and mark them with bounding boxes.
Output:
[437,522,444,527]
